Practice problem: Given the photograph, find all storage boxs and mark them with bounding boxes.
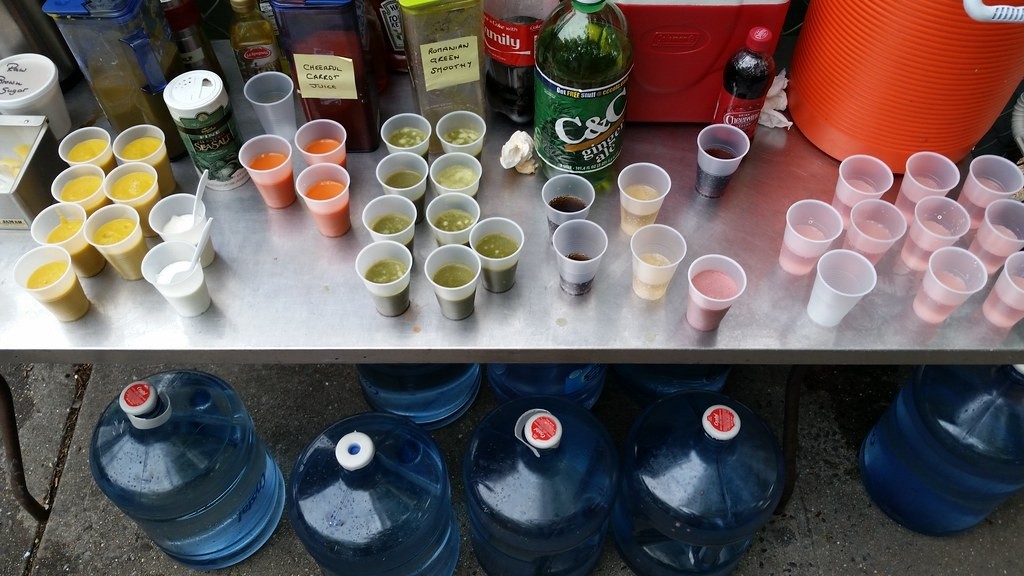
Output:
[1,116,65,230]
[614,0,791,123]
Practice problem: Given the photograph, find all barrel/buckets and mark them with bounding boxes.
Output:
[786,0,1024,174]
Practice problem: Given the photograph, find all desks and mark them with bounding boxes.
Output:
[0,38,1024,520]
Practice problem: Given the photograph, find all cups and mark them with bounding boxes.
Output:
[243,72,296,142]
[356,110,481,320]
[779,151,1024,328]
[238,119,351,237]
[696,124,750,198]
[630,224,687,301]
[13,124,214,322]
[686,254,747,331]
[553,219,608,296]
[0,53,71,140]
[469,217,525,293]
[618,162,671,236]
[542,174,595,246]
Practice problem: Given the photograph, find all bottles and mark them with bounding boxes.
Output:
[711,27,776,158]
[859,364,1024,536]
[89,370,285,570]
[463,363,618,576]
[619,363,790,576]
[160,0,282,191]
[357,363,482,431]
[483,0,631,188]
[287,413,460,576]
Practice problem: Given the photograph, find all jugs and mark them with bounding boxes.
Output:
[42,0,187,162]
[267,0,486,153]
